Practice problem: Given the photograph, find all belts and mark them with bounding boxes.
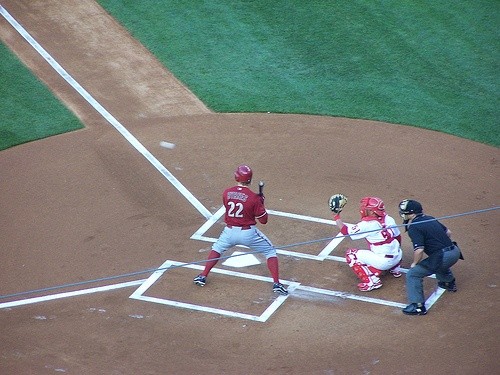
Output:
[227,224,250,231]
[385,255,393,258]
[442,245,454,252]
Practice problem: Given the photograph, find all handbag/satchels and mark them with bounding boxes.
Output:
[423,252,443,273]
[452,242,464,260]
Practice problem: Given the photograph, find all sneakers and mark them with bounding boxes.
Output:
[358,279,382,292]
[273,283,287,296]
[389,268,402,278]
[438,281,456,291]
[193,275,206,286]
[402,302,426,314]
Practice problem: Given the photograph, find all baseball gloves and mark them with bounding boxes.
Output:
[328,194,347,214]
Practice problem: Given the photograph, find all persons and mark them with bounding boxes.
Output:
[193,165,288,297]
[398,198,461,316]
[329,196,403,291]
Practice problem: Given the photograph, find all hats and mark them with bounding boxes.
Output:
[406,200,423,214]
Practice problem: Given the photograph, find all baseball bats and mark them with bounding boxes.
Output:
[258,181,265,196]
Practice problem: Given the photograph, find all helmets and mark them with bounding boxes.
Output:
[368,197,387,216]
[234,165,252,182]
